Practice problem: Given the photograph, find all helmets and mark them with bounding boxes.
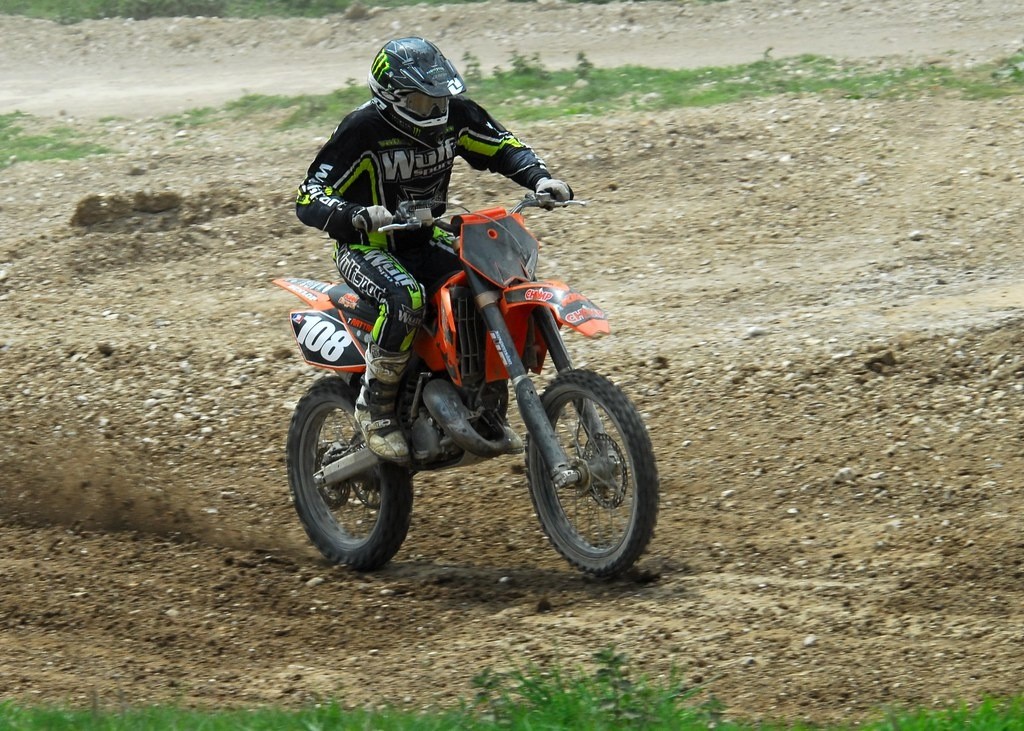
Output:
[367,36,467,149]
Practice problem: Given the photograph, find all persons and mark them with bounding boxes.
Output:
[297,35,573,463]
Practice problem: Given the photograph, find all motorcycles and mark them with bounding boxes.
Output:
[271,187,659,580]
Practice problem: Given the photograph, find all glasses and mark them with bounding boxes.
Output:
[402,90,449,117]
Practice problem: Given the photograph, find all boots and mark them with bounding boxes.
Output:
[354,327,412,460]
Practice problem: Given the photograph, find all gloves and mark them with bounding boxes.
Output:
[535,175,575,211]
[351,205,393,232]
